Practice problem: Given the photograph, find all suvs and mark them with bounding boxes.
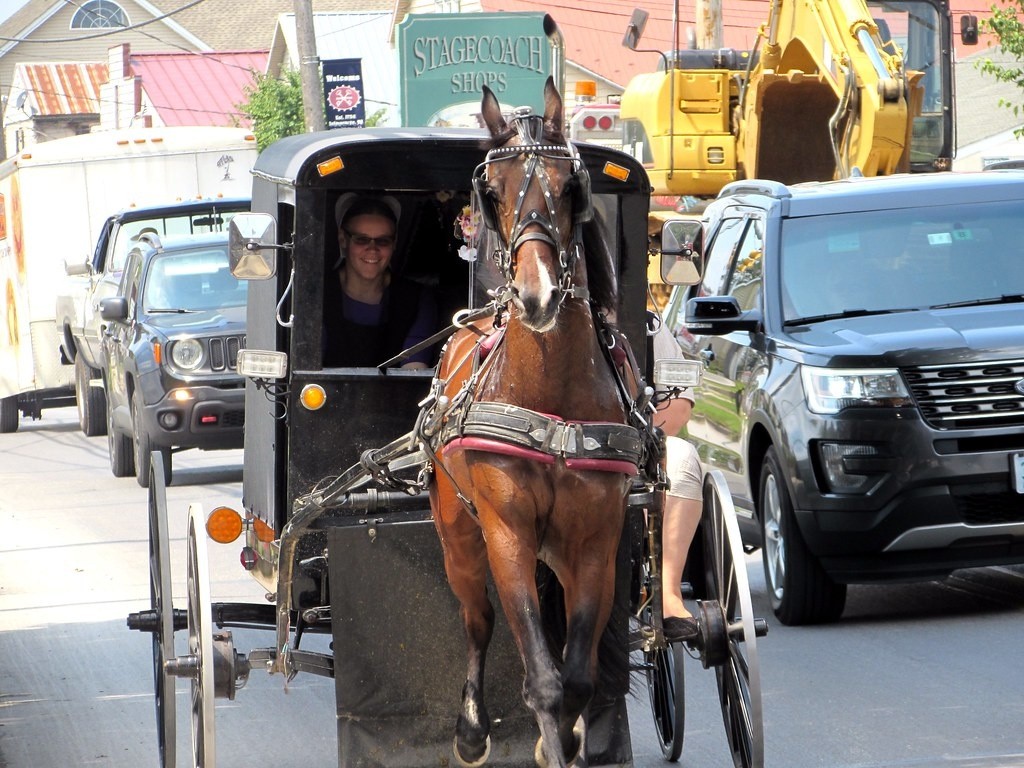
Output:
[98,222,256,489]
[641,158,1024,629]
[58,194,260,440]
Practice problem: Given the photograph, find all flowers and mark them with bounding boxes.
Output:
[457,204,482,263]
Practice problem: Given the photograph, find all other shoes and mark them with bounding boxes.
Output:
[661,615,698,642]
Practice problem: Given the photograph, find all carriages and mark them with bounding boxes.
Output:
[126,10,774,768]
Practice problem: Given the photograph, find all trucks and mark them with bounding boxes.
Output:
[1,123,258,436]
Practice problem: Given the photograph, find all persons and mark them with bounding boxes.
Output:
[645,309,703,640]
[322,194,434,370]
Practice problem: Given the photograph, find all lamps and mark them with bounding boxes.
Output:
[236,350,288,378]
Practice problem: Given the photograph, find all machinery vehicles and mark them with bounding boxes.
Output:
[613,0,934,322]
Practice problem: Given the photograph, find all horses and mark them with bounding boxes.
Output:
[414,75,666,768]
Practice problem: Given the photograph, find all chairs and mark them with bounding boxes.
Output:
[208,267,239,303]
[167,272,202,307]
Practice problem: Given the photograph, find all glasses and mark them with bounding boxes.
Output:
[343,228,396,247]
